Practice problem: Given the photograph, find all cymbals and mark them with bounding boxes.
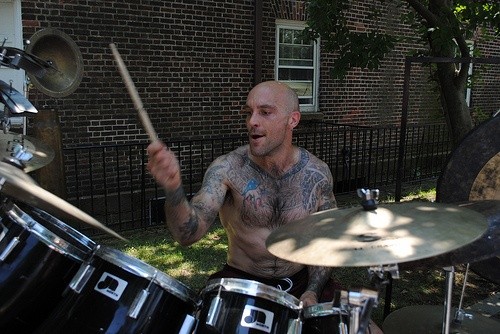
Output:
[0,130,55,173]
[0,162,131,243]
[382,303,500,334]
[22,28,84,99]
[259,202,462,268]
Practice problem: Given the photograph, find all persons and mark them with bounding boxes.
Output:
[145,79,385,334]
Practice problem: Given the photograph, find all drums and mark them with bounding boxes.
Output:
[299,301,350,334]
[0,199,98,334]
[434,116,500,284]
[195,277,303,334]
[58,243,195,334]
